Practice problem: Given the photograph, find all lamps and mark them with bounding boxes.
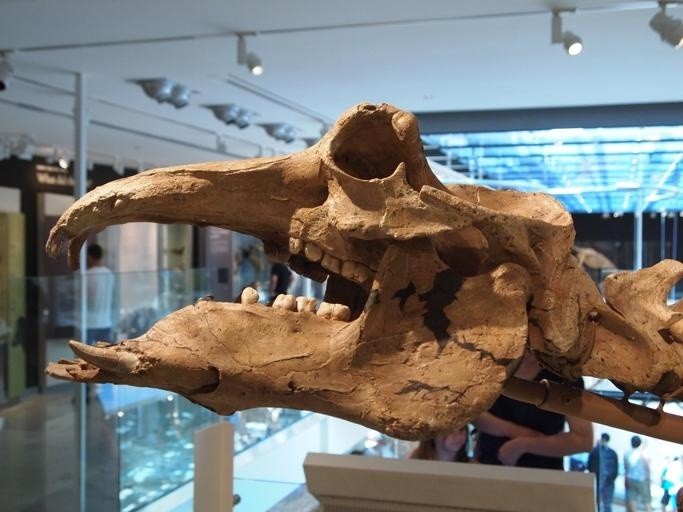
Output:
[551,11,581,54]
[648,2,683,50]
[125,76,323,147]
[237,37,264,74]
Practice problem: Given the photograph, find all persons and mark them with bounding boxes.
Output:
[233,249,259,304]
[264,258,294,310]
[655,455,682,512]
[474,344,593,471]
[567,449,590,471]
[407,421,480,465]
[624,434,657,512]
[586,433,619,512]
[70,243,117,405]
